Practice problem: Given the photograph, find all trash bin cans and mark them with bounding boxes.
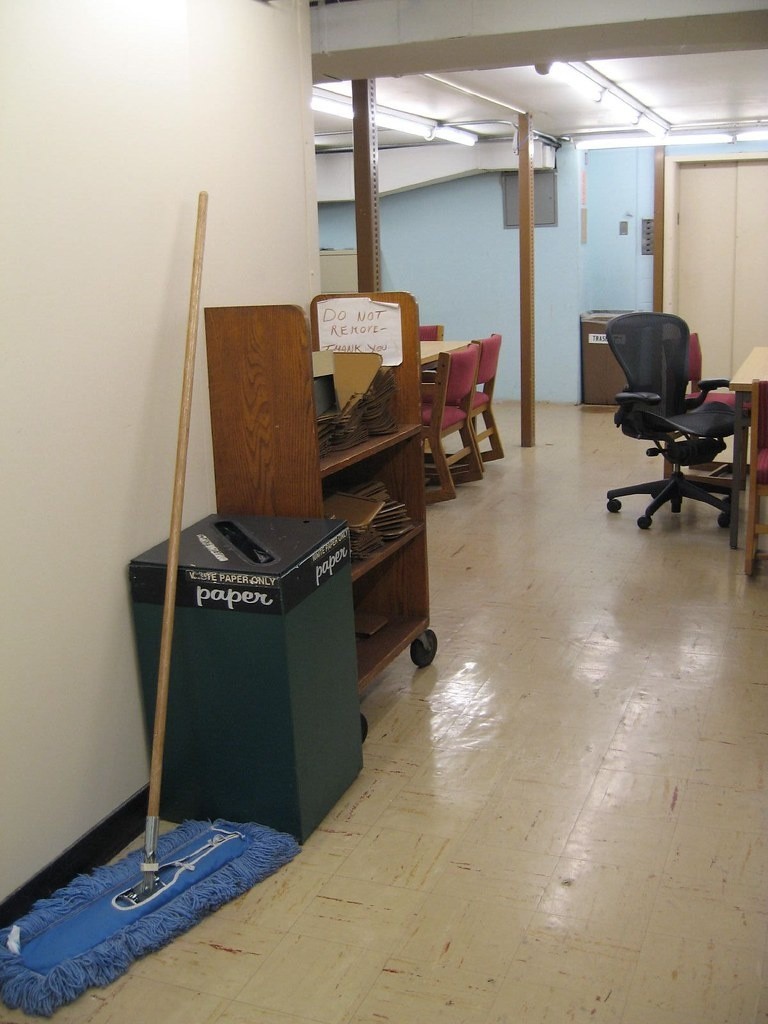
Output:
[127,513,363,845]
[580,309,639,405]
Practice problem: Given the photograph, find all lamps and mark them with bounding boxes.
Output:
[310,62,768,150]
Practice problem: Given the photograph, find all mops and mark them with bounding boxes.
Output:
[0,185,300,1016]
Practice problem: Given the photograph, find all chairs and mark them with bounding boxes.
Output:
[419,324,505,506]
[744,379,768,575]
[604,311,735,529]
[663,332,751,491]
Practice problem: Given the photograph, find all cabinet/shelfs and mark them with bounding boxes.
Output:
[204,290,438,745]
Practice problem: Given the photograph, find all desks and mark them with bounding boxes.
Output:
[420,340,471,365]
[728,347,768,550]
